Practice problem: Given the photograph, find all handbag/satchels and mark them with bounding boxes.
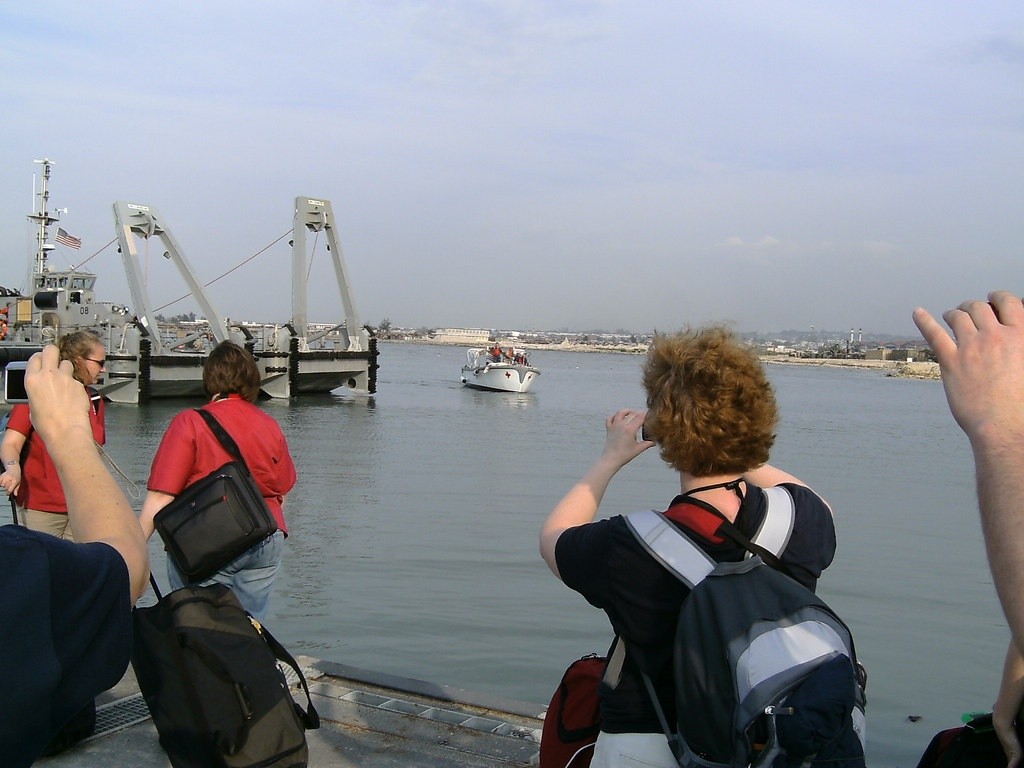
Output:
[0,423,34,475]
[540,654,609,768]
[0,524,135,768]
[152,409,278,592]
[916,713,1016,768]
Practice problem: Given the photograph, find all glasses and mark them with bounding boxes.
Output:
[81,355,105,369]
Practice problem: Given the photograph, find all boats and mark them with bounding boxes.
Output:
[460,346,541,394]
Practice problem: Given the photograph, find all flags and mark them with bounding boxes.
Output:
[55,228,82,251]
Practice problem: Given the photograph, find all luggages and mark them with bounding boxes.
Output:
[127,571,321,768]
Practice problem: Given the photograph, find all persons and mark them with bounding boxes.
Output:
[138,340,296,623]
[0,345,150,768]
[539,325,837,768]
[0,302,11,341]
[198,327,213,340]
[0,332,107,545]
[912,290,1024,768]
[490,341,532,367]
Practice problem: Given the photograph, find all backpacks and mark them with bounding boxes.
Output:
[605,484,867,768]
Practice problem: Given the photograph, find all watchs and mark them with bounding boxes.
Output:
[5,460,18,470]
